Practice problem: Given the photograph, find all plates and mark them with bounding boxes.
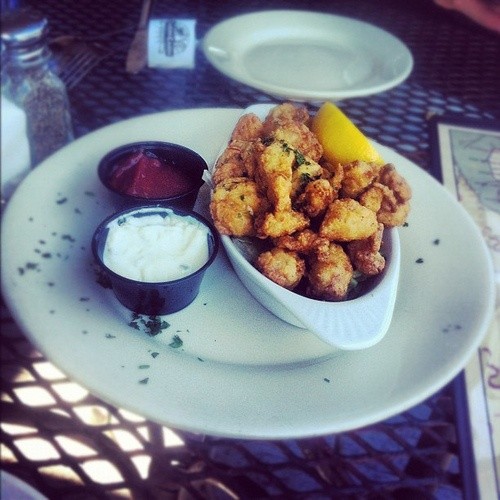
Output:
[201,9,414,104]
[211,103,399,351]
[2,108,498,441]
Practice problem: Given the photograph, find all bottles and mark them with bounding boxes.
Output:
[0,9,73,200]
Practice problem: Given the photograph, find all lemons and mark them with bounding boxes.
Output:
[314,101,383,166]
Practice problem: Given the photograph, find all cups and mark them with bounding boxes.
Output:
[92,202,220,317]
[98,141,209,212]
